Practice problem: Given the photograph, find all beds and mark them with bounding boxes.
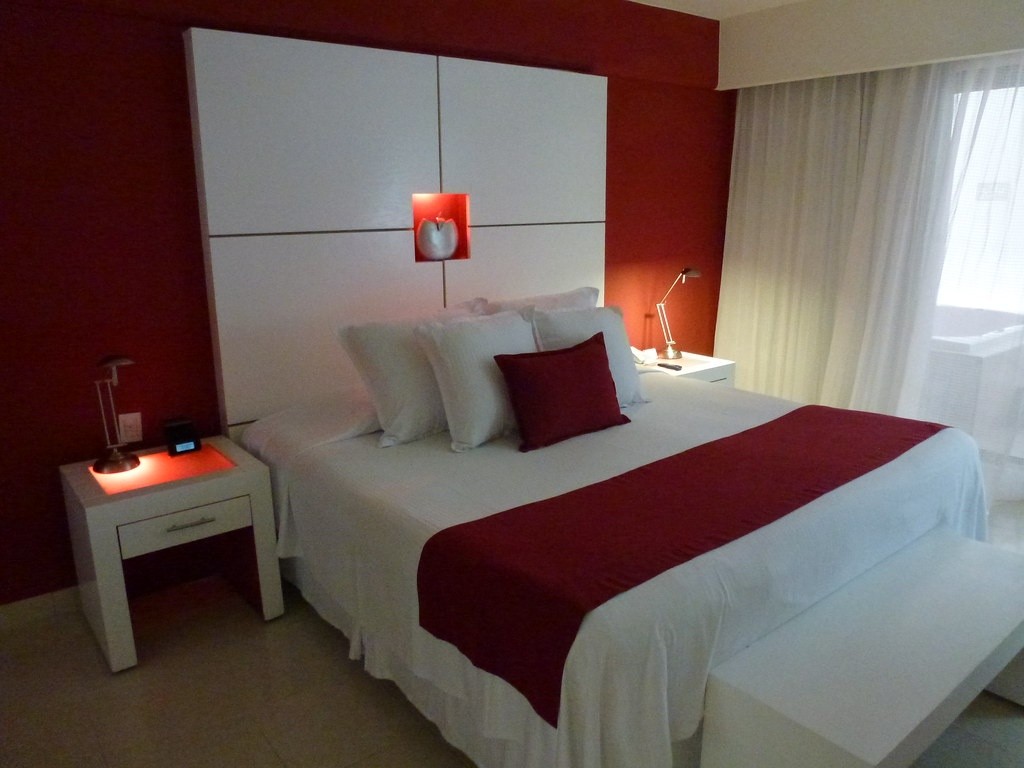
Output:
[240,344,978,768]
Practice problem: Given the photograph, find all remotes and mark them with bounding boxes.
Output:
[658,362,683,371]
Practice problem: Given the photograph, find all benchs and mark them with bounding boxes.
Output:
[701,525,1024,768]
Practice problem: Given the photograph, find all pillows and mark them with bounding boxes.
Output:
[490,286,599,316]
[330,296,488,448]
[493,332,631,454]
[413,312,531,453]
[533,306,649,406]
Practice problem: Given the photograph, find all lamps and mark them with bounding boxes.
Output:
[656,266,702,359]
[92,357,140,476]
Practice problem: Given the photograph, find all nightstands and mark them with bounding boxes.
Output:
[58,433,284,673]
[640,349,736,390]
